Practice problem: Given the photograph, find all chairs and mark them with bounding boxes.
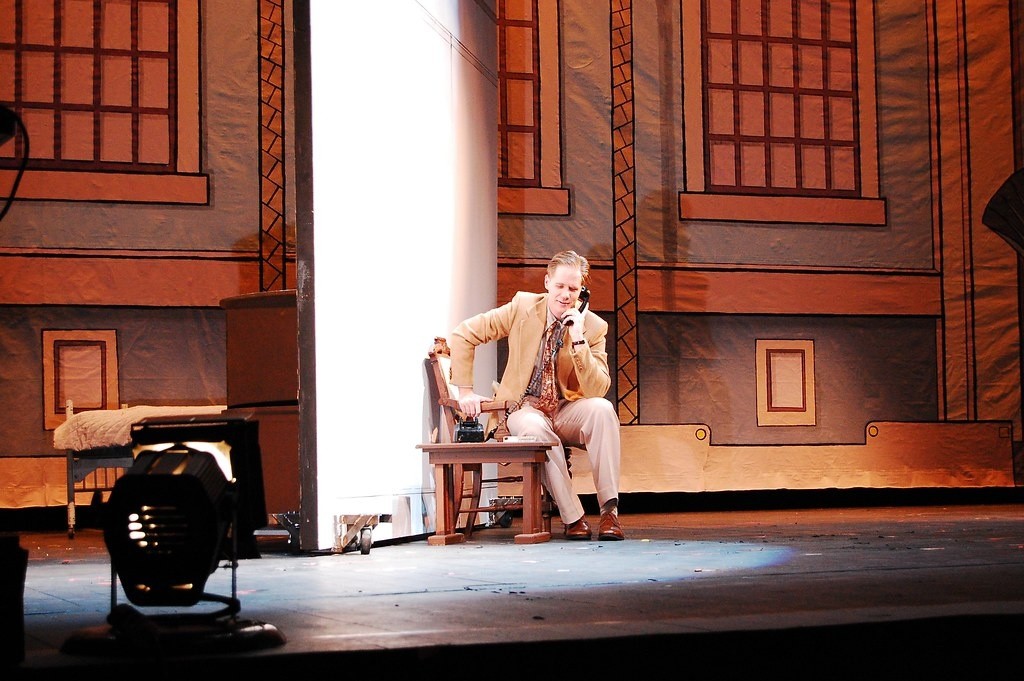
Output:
[427,335,572,542]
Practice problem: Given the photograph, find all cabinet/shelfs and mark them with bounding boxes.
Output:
[220,288,300,514]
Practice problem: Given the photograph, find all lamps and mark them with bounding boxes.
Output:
[102,448,242,619]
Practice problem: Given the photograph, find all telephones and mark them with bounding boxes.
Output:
[558,286,590,327]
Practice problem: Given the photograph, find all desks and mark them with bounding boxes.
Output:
[416,440,559,545]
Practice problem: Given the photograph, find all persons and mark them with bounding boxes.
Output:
[450,251,623,541]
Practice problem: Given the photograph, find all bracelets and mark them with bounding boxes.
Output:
[572,339,586,349]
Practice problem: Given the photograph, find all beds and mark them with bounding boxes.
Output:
[52,399,236,540]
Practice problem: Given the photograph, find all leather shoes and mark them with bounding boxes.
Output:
[598,512,625,541]
[564,518,592,541]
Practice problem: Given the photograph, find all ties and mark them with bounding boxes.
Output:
[534,322,560,411]
